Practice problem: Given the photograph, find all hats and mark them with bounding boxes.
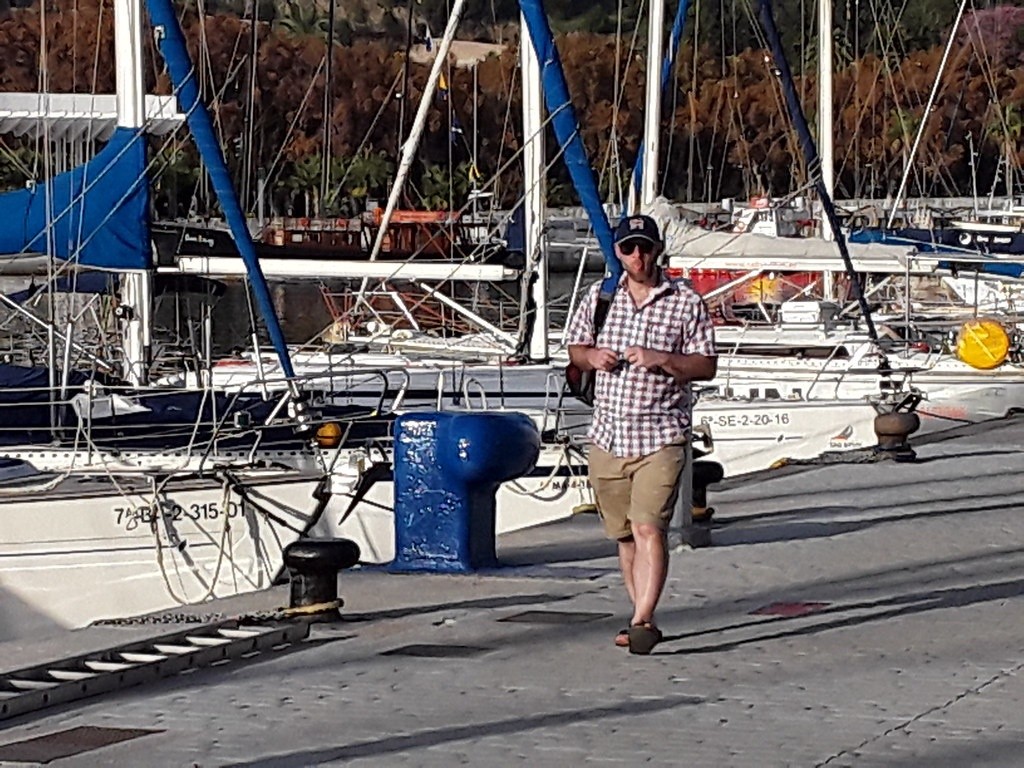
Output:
[613,214,659,246]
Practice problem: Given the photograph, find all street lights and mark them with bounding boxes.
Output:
[705,166,714,206]
[865,164,875,201]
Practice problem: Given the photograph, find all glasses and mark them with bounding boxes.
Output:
[618,238,655,255]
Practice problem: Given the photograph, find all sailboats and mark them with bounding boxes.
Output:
[0,0,1024,645]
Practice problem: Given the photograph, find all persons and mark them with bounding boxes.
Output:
[566,215,717,657]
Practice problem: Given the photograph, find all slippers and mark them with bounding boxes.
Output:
[629,620,662,655]
[615,627,629,647]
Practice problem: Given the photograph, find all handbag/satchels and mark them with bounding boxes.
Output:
[564,278,617,408]
